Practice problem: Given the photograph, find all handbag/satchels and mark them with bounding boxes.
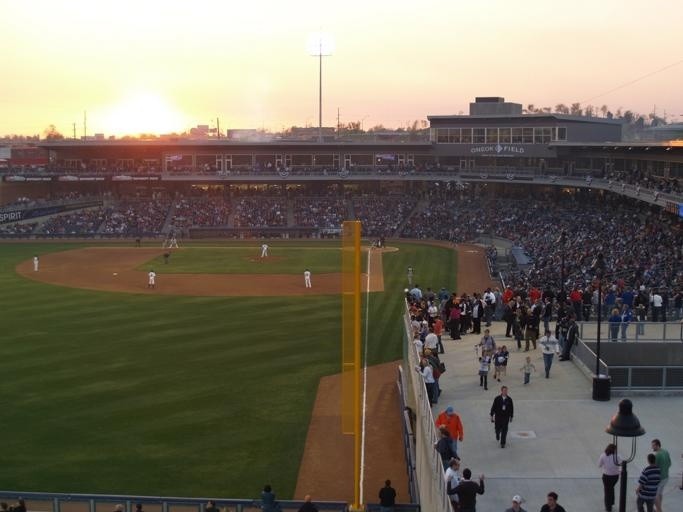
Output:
[433,369,441,379]
[438,363,445,373]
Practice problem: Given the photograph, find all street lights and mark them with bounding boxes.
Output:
[588,251,613,401]
[604,397,644,512]
[553,228,568,340]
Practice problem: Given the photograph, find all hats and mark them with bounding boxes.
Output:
[446,405,454,416]
[512,494,521,504]
[449,458,461,466]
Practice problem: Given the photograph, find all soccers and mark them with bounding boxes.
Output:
[112,272,117,276]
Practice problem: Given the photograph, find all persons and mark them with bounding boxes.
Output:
[168,237,179,249]
[260,243,269,258]
[205,500,220,511]
[1,502,11,512]
[33,254,39,273]
[379,479,398,512]
[0,158,683,239]
[370,234,385,258]
[14,496,27,511]
[406,240,683,511]
[261,484,276,508]
[148,271,156,289]
[9,504,17,512]
[135,503,146,512]
[303,269,312,289]
[298,495,320,512]
[113,504,127,512]
